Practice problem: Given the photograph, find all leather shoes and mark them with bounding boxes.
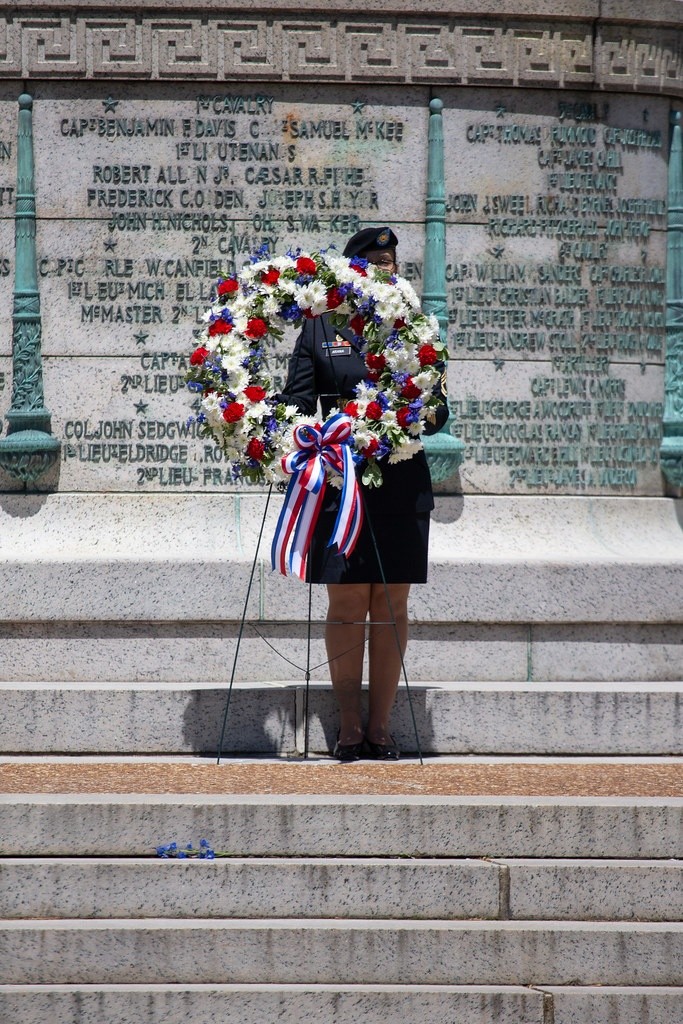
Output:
[364,727,400,761]
[334,727,364,760]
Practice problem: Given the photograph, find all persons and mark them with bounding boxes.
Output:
[270,228,450,759]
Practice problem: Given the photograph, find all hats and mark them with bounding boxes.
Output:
[342,227,398,259]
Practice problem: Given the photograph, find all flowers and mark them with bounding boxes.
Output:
[186,257,445,487]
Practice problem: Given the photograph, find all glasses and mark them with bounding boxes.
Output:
[368,260,395,271]
